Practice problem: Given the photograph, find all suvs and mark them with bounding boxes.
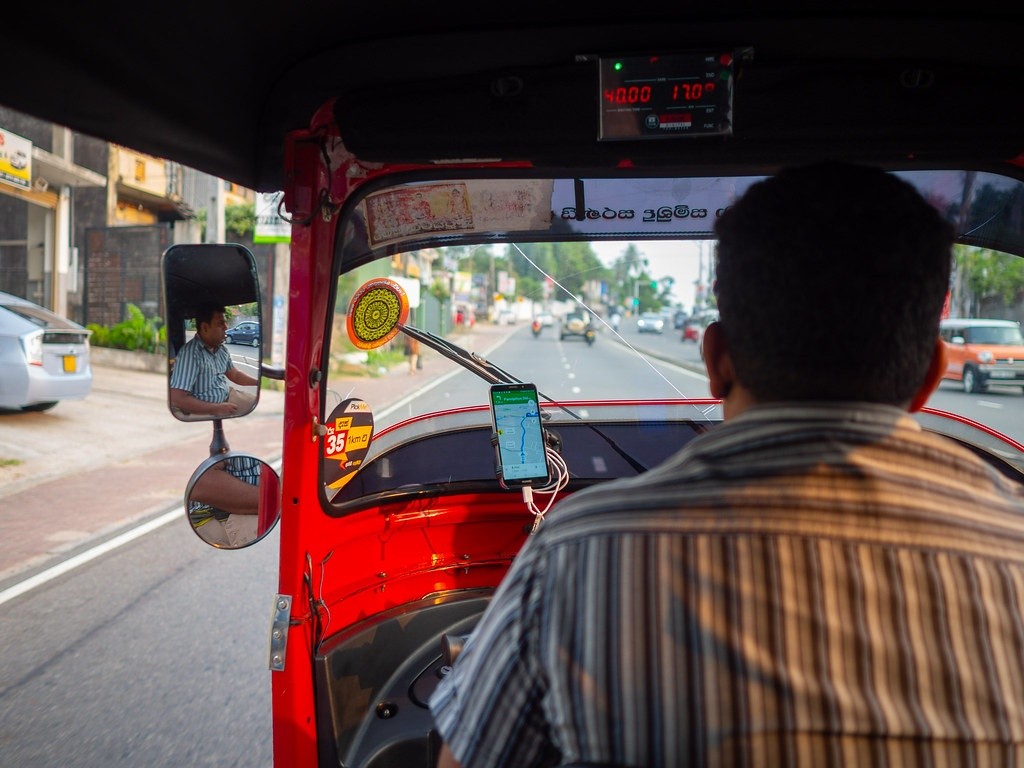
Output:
[560,308,594,340]
[224,321,260,346]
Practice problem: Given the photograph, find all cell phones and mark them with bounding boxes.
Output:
[489,383,550,490]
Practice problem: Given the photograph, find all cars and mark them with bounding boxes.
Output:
[538,313,554,326]
[0,292,94,414]
[638,311,664,333]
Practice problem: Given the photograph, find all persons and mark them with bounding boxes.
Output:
[170,302,258,417]
[190,457,263,546]
[536,312,542,323]
[611,312,620,326]
[430,161,1023,768]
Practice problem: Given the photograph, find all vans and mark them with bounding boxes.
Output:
[939,318,1024,392]
